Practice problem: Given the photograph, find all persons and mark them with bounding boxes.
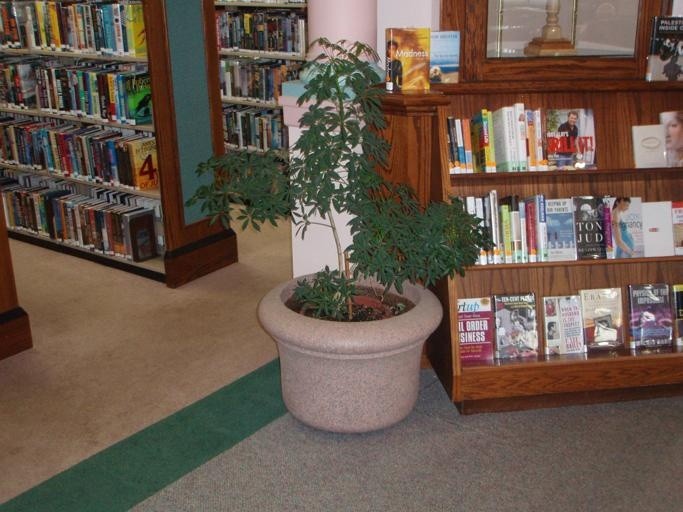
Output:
[386,40,402,92]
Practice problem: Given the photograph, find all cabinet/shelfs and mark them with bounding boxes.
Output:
[211,0,291,168]
[2,0,176,286]
[439,85,681,416]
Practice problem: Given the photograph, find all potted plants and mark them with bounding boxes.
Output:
[184,37,496,436]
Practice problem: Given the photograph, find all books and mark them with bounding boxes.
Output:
[459,189,682,267]
[1,1,165,264]
[385,26,430,91]
[430,30,460,85]
[446,101,599,174]
[645,17,683,82]
[216,5,306,198]
[458,281,683,362]
[631,111,682,169]
